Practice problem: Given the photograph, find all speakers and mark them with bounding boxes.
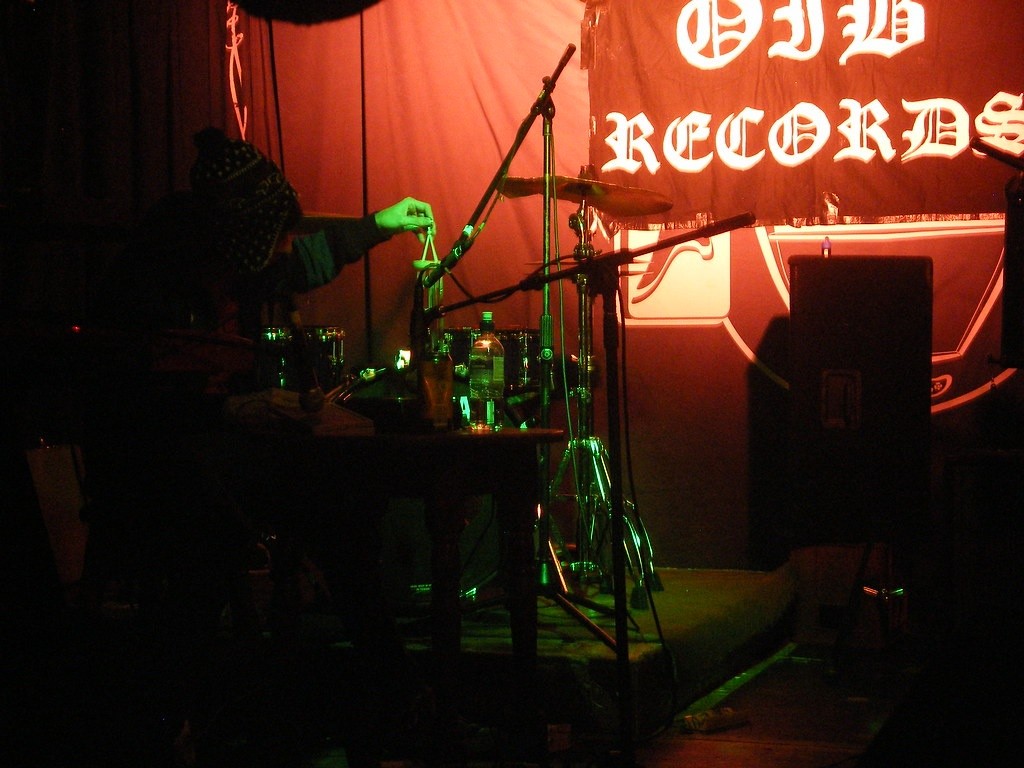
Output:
[788,254,932,546]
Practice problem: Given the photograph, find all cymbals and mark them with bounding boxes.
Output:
[497,174,674,215]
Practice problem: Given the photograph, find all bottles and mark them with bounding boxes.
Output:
[467,311,506,433]
[821,237,831,256]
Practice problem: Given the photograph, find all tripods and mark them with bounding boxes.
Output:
[546,197,663,602]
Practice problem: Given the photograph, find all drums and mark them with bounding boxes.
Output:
[441,328,545,392]
[261,323,346,393]
[350,393,421,431]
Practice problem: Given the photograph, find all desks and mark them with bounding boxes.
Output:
[314,426,568,685]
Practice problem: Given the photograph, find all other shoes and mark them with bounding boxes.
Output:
[359,675,507,755]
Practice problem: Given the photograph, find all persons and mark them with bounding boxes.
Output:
[79,127,494,766]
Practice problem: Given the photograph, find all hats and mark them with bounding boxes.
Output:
[189,127,296,272]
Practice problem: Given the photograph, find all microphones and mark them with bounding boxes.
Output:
[424,239,474,289]
[411,277,432,362]
[286,304,324,411]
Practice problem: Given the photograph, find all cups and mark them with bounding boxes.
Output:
[416,351,453,426]
